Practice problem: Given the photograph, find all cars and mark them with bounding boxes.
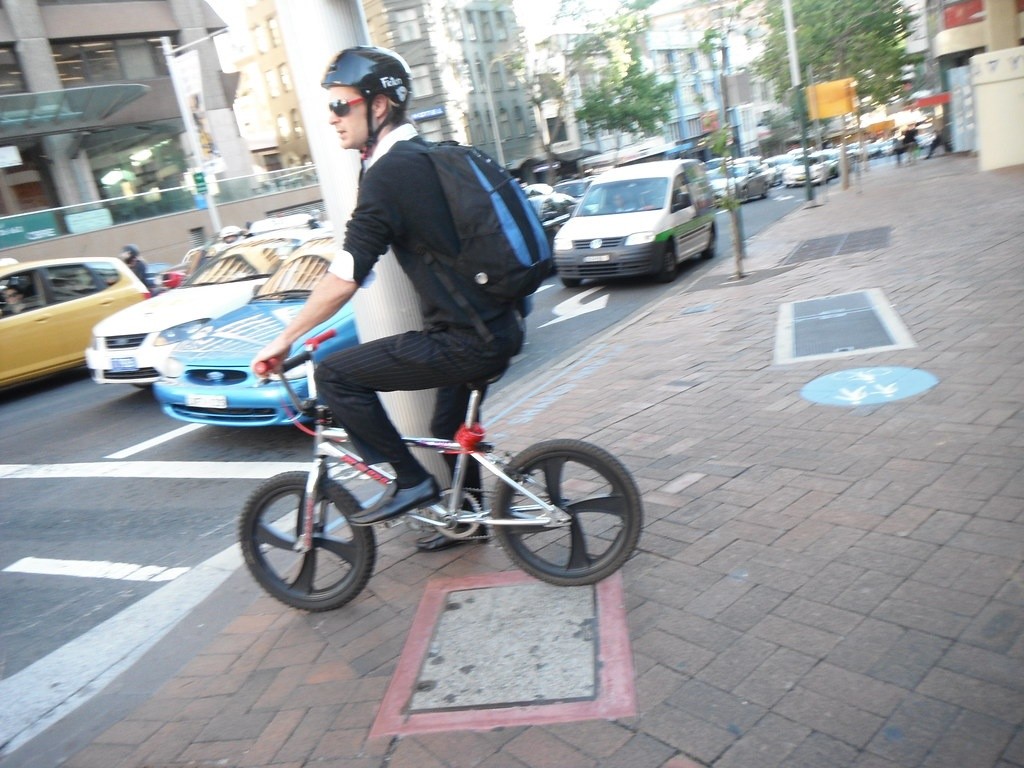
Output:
[764,132,934,187]
[522,175,598,278]
[83,227,327,390]
[151,232,361,431]
[700,156,729,180]
[0,257,152,391]
[708,163,769,207]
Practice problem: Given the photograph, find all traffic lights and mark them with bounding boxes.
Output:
[192,173,208,193]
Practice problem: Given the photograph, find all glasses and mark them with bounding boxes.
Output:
[328,97,366,118]
[2,292,19,298]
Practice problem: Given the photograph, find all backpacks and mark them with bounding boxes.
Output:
[387,140,553,344]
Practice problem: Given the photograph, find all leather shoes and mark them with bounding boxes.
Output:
[347,475,442,527]
[416,525,488,552]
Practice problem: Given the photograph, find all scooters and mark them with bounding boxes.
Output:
[143,272,186,297]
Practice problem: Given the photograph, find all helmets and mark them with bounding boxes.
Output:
[320,45,413,160]
[119,244,140,264]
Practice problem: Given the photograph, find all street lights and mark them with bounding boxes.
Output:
[167,25,230,235]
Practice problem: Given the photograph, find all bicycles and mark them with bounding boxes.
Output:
[239,328,642,613]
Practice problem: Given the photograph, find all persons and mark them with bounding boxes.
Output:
[251,46,526,552]
[118,244,146,285]
[0,285,39,320]
[893,122,950,164]
[603,192,637,213]
[220,226,242,244]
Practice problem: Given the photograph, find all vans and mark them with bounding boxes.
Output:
[551,156,718,288]
